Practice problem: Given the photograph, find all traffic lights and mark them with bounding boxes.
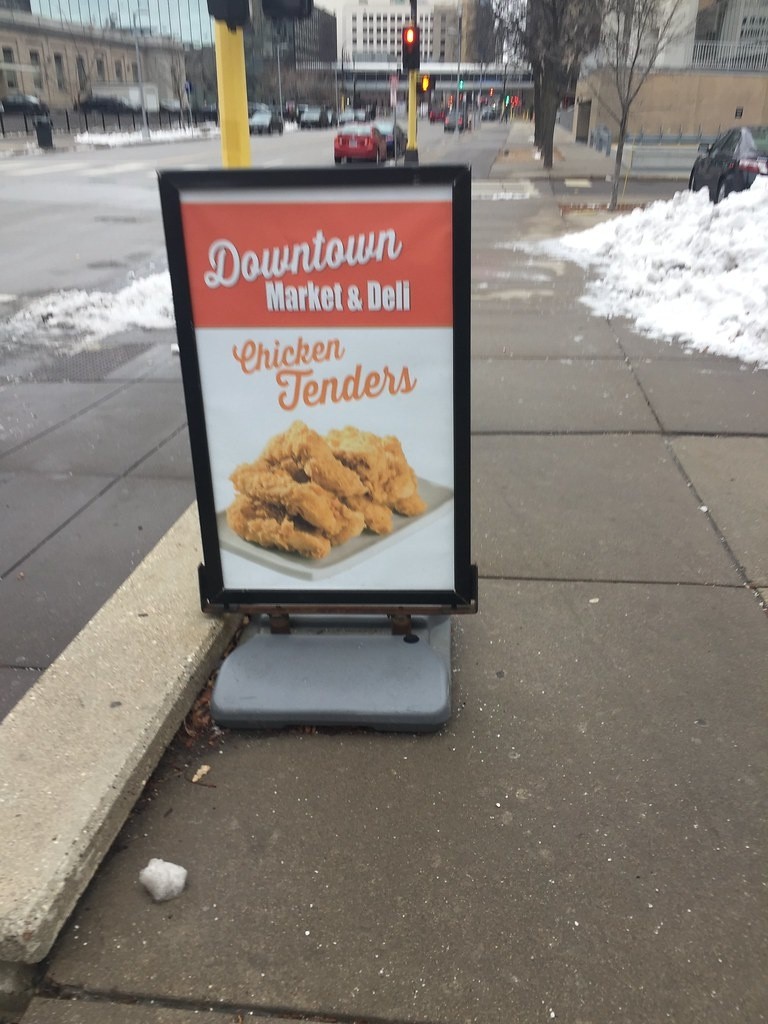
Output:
[416,74,433,93]
[402,26,420,70]
[446,79,520,108]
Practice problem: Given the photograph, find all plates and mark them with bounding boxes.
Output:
[215,476,454,579]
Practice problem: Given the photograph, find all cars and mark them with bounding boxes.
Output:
[689,110,768,204]
[248,109,285,136]
[429,104,448,123]
[482,109,499,122]
[444,112,469,131]
[292,99,375,136]
[372,122,409,158]
[335,125,388,164]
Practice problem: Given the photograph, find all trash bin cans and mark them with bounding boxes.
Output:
[32,118,55,150]
